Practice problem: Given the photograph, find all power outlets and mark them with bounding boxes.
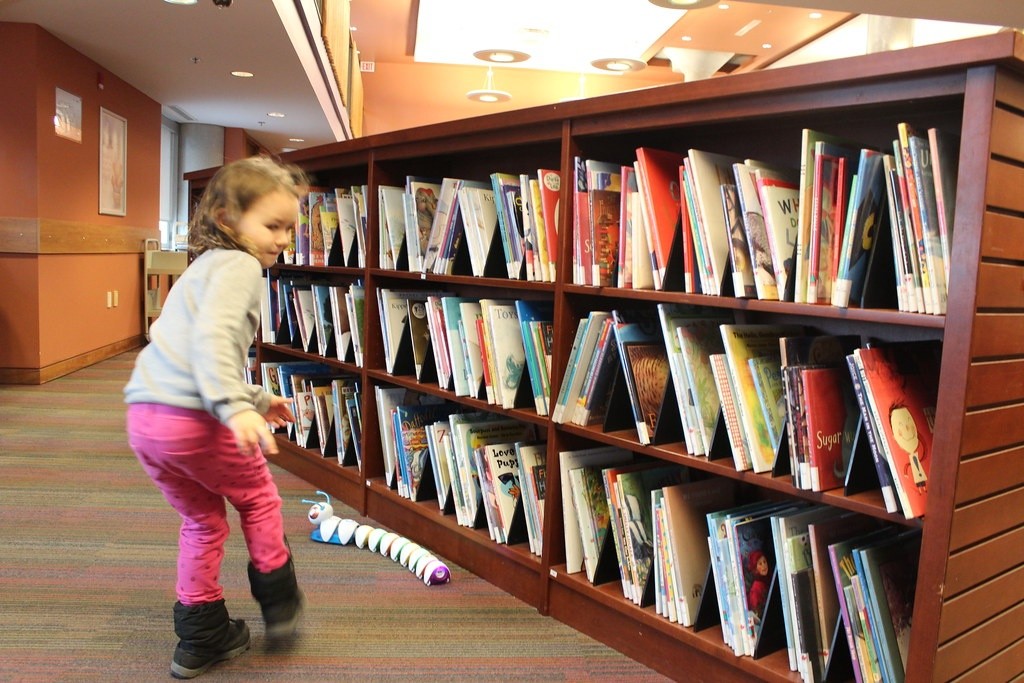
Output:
[107,292,112,308]
[114,291,118,307]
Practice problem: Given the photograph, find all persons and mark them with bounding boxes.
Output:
[123,156,309,679]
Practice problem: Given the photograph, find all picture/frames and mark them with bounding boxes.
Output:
[99,106,127,217]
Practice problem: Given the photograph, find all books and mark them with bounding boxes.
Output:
[377,123,953,683]
[244,183,368,473]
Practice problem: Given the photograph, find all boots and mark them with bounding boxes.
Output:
[170,600,251,679]
[246,552,306,640]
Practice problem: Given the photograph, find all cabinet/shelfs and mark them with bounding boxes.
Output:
[143,239,187,342]
[183,27,1024,683]
